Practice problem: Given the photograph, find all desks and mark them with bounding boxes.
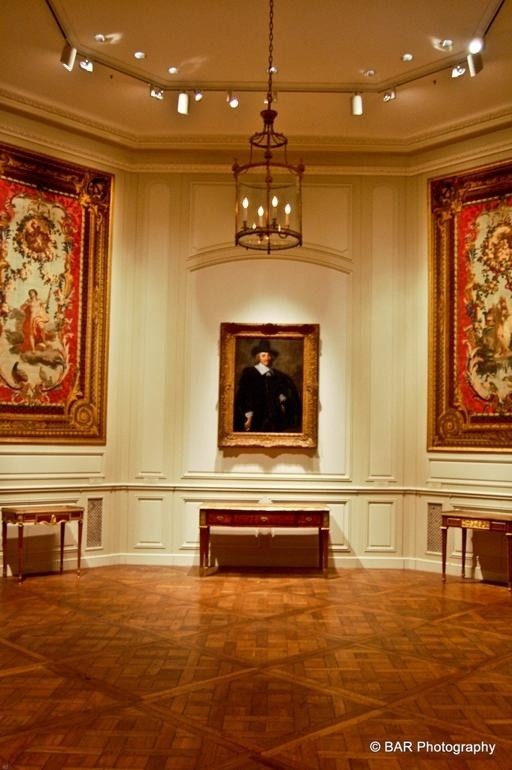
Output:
[198,508,330,578]
[440,512,512,593]
[0,505,84,587]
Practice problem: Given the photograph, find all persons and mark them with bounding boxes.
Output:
[236,340,302,433]
[21,288,53,353]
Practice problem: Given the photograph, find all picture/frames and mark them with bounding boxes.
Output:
[423,154,512,454]
[217,320,321,450]
[0,138,117,447]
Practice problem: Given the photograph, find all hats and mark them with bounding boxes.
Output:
[252,340,278,356]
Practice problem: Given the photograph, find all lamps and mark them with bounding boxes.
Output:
[229,1,305,256]
[58,32,486,120]
